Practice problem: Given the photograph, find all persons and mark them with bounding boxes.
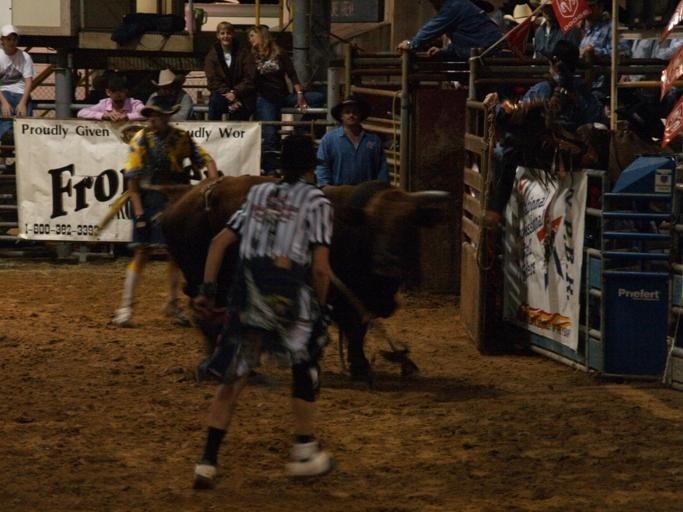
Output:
[0,0,632,325]
[193,134,333,490]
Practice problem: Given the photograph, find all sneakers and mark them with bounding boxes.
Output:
[191,463,218,485]
[114,303,135,325]
[281,440,334,480]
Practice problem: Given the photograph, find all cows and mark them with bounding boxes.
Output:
[152,173,450,383]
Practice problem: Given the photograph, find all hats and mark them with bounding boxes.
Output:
[0,24,18,38]
[149,67,186,89]
[538,39,595,73]
[330,92,369,123]
[501,3,539,27]
[139,91,183,116]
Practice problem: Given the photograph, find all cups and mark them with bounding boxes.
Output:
[195,7,209,31]
